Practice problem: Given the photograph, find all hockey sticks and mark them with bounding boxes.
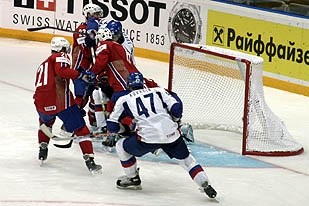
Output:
[54,132,75,148]
[40,123,137,141]
[27,26,87,35]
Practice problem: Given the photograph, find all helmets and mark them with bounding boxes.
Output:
[83,4,102,18]
[127,72,144,89]
[51,37,70,52]
[96,28,112,41]
[106,19,122,35]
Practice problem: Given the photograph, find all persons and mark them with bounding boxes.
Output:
[101,71,217,199]
[71,4,158,139]
[33,36,103,176]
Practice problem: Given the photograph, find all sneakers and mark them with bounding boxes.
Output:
[117,168,141,190]
[60,124,69,136]
[39,147,48,166]
[202,182,220,203]
[90,125,118,143]
[83,155,102,175]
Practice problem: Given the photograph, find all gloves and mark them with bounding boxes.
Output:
[78,71,95,83]
